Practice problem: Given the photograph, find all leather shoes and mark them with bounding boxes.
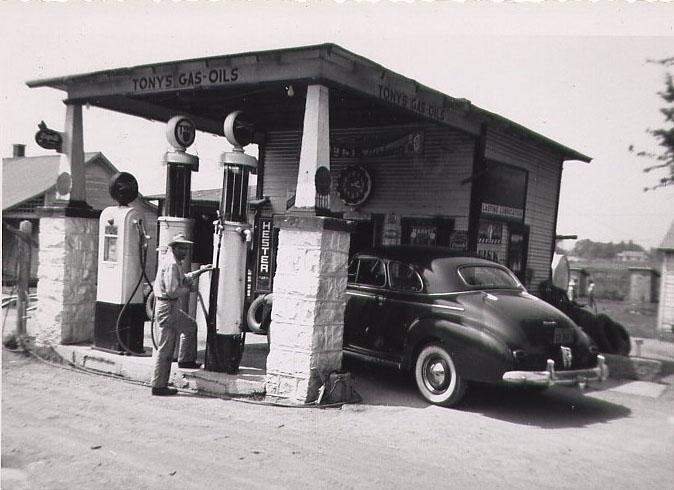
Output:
[177,361,200,368]
[151,387,178,396]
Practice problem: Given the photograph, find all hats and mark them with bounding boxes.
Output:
[168,234,195,246]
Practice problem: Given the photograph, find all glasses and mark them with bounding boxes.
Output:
[175,246,189,250]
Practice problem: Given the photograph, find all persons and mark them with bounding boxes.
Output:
[586,278,596,308]
[150,233,214,396]
[567,277,578,302]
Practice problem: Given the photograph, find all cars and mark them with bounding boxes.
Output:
[260,245,611,406]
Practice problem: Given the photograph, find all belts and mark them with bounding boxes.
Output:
[157,296,178,301]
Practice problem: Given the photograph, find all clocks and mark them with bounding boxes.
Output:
[337,162,374,208]
